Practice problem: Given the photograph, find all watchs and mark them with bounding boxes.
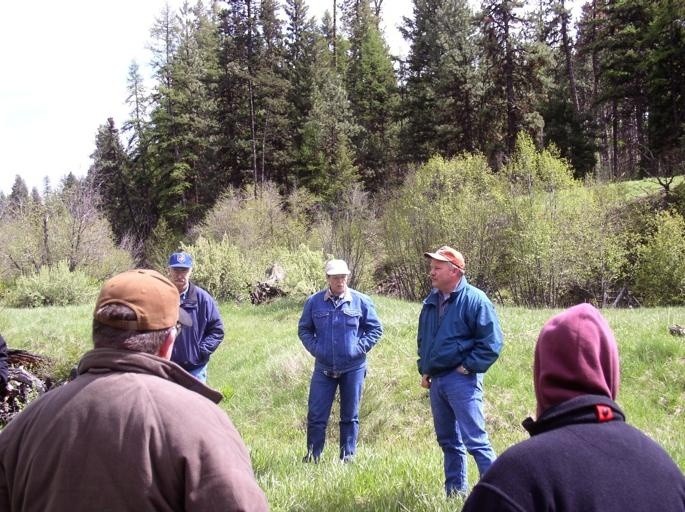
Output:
[461,368,471,374]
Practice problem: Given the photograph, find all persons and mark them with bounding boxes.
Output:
[0,268,270,512]
[416,246,504,496]
[0,333,9,400]
[298,259,383,463]
[167,253,225,385]
[462,302,685,512]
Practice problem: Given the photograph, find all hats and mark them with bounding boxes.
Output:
[94,269,193,330]
[325,259,350,276]
[169,253,193,269]
[424,245,465,269]
